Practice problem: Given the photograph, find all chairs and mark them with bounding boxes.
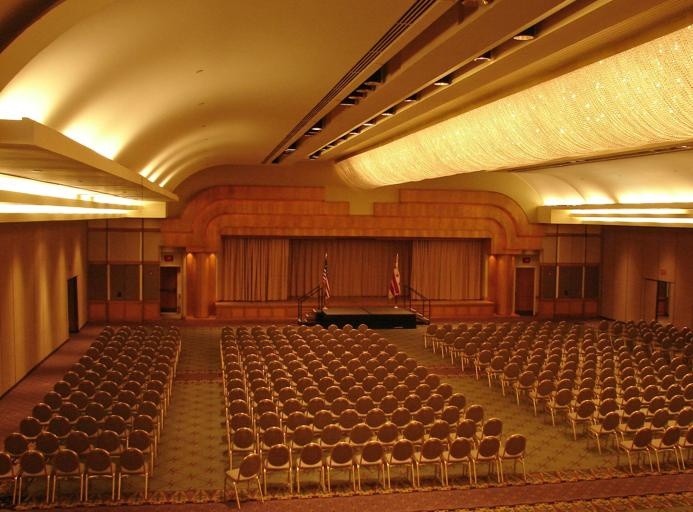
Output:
[0,325,182,505]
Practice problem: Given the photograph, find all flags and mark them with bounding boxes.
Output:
[388,262,401,299]
[321,264,331,301]
